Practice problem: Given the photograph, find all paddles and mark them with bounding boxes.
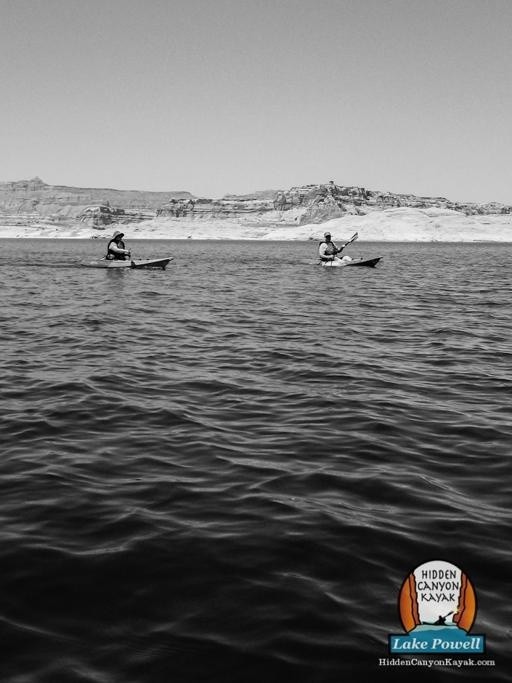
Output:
[333,232,358,255]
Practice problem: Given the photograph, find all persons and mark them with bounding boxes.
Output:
[318,232,353,265]
[106,231,131,261]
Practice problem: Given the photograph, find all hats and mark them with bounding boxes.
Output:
[324,232,331,237]
[113,231,124,239]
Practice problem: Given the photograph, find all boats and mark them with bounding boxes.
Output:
[81,254,176,270]
[320,255,386,267]
